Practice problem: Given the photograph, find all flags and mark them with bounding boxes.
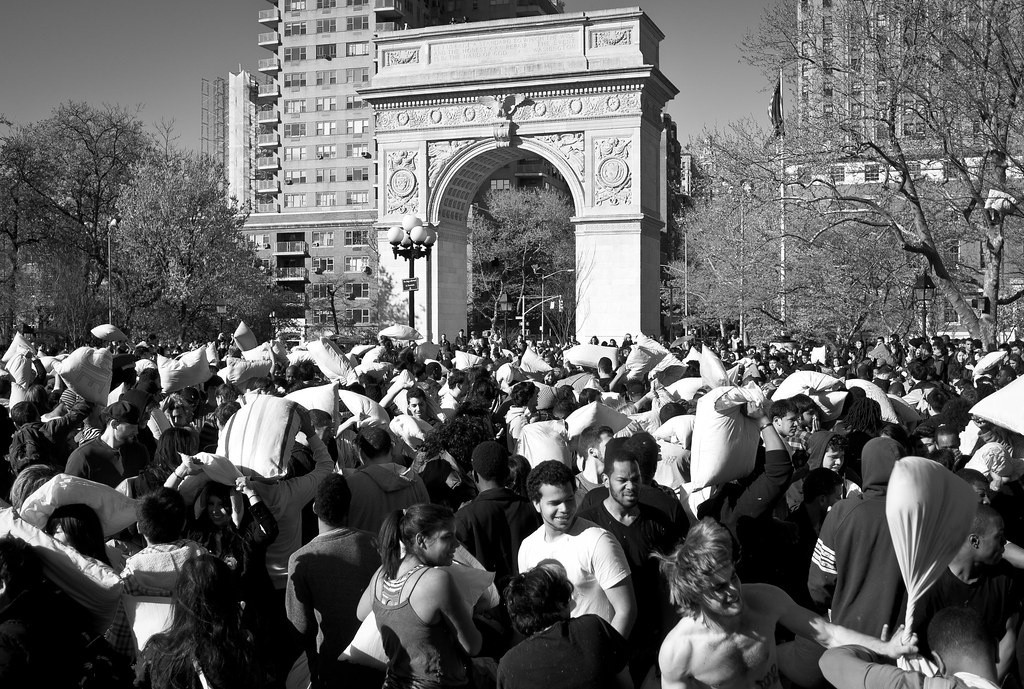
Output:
[766,79,786,136]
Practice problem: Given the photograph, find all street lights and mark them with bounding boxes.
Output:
[541,269,575,342]
[215,294,227,333]
[913,270,936,335]
[107,218,118,326]
[728,178,755,338]
[383,214,438,348]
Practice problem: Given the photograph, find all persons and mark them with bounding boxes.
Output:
[1,328,1023,689]
[659,516,919,689]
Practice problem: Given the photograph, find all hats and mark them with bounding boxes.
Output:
[909,338,923,348]
[101,400,139,426]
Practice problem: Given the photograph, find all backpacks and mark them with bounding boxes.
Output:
[10,422,48,476]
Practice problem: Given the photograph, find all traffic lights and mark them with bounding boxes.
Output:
[559,298,564,313]
